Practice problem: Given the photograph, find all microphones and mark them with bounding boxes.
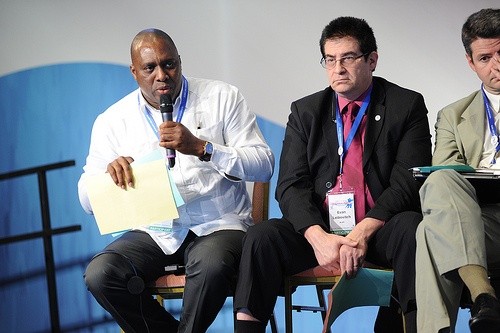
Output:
[160,93,176,168]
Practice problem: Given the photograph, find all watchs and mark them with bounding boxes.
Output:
[199,141,213,162]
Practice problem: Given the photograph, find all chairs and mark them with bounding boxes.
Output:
[144,182,342,333]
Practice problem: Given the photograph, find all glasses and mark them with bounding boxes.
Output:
[320,52,370,69]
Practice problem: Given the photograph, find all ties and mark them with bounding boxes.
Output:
[334,102,365,226]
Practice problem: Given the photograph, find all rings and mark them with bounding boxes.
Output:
[353,263,359,268]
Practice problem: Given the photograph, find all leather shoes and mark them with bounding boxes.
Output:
[469,294,499,333]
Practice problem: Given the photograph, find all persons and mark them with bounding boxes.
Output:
[415,9,500,333]
[232,17,432,333]
[77,28,274,333]
